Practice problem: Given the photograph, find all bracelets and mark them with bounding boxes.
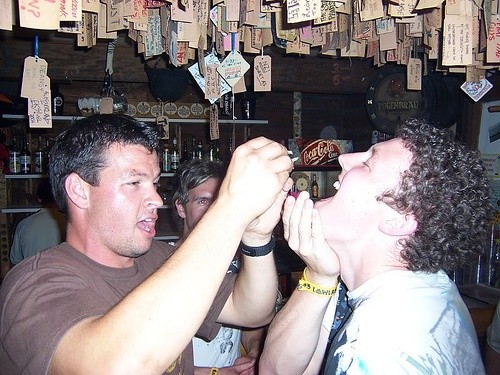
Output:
[297,267,339,296]
[239,234,276,257]
[209,367,219,375]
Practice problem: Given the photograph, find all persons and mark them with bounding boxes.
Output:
[0,113,295,375]
[258,118,495,375]
[9,177,67,266]
[170,157,268,375]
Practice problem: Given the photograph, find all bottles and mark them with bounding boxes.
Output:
[34,135,45,174]
[8,132,21,174]
[312,175,318,198]
[162,132,232,173]
[20,137,31,174]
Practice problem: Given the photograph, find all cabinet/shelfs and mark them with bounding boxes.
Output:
[0,112,269,240]
